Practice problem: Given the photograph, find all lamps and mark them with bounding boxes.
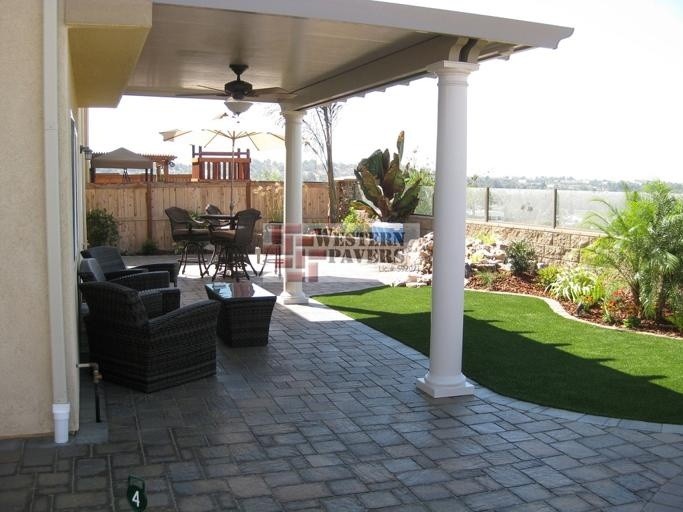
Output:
[80,144,93,160]
[223,100,253,116]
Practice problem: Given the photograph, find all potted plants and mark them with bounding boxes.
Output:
[354,131,422,262]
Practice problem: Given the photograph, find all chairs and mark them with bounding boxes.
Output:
[80,258,170,291]
[80,246,179,287]
[259,223,282,280]
[79,281,222,394]
[164,204,262,283]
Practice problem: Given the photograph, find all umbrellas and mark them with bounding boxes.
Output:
[151,106,310,218]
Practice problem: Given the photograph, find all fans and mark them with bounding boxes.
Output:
[176,64,298,100]
